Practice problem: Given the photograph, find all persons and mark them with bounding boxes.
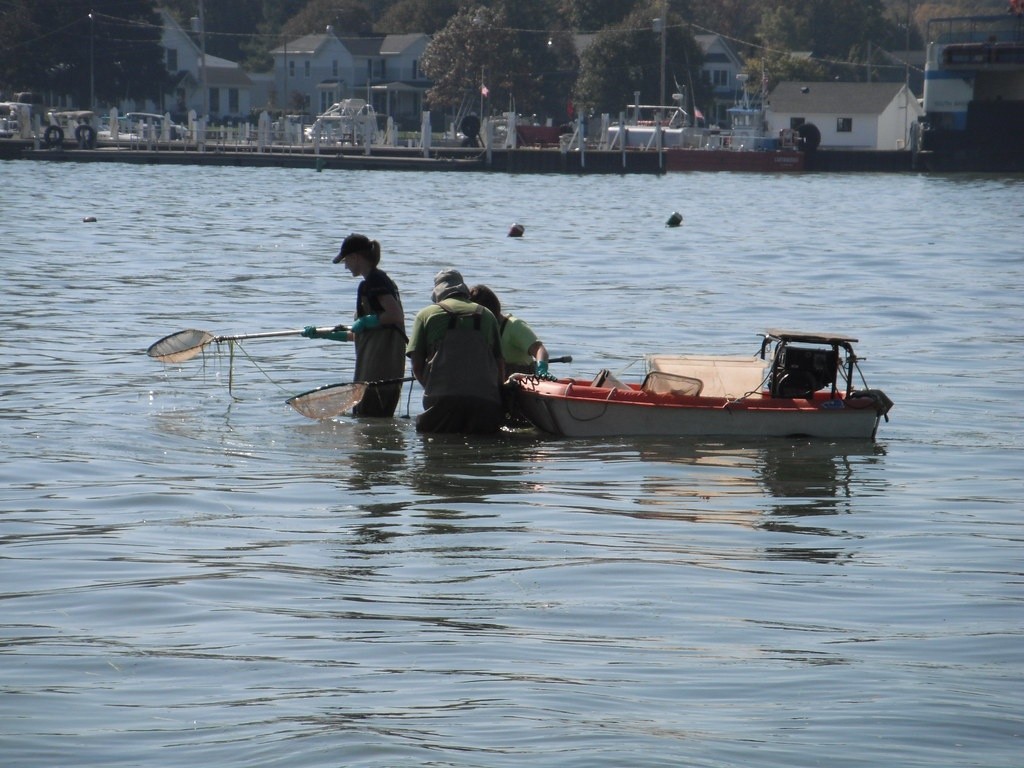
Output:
[468,284,556,384]
[298,233,404,417]
[407,268,509,435]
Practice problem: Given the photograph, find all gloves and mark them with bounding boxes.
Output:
[530,361,557,380]
[350,314,380,334]
[300,325,347,342]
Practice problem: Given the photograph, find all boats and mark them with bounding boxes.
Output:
[503,332,894,442]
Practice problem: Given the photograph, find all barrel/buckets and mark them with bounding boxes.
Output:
[590,367,633,392]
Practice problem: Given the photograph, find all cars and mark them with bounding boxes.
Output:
[124,113,187,140]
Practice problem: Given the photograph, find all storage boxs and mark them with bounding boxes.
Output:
[647,353,771,398]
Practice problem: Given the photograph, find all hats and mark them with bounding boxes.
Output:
[430,270,471,302]
[332,233,369,264]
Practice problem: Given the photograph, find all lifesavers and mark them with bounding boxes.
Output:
[72,122,99,148]
[44,123,65,147]
[460,112,482,140]
